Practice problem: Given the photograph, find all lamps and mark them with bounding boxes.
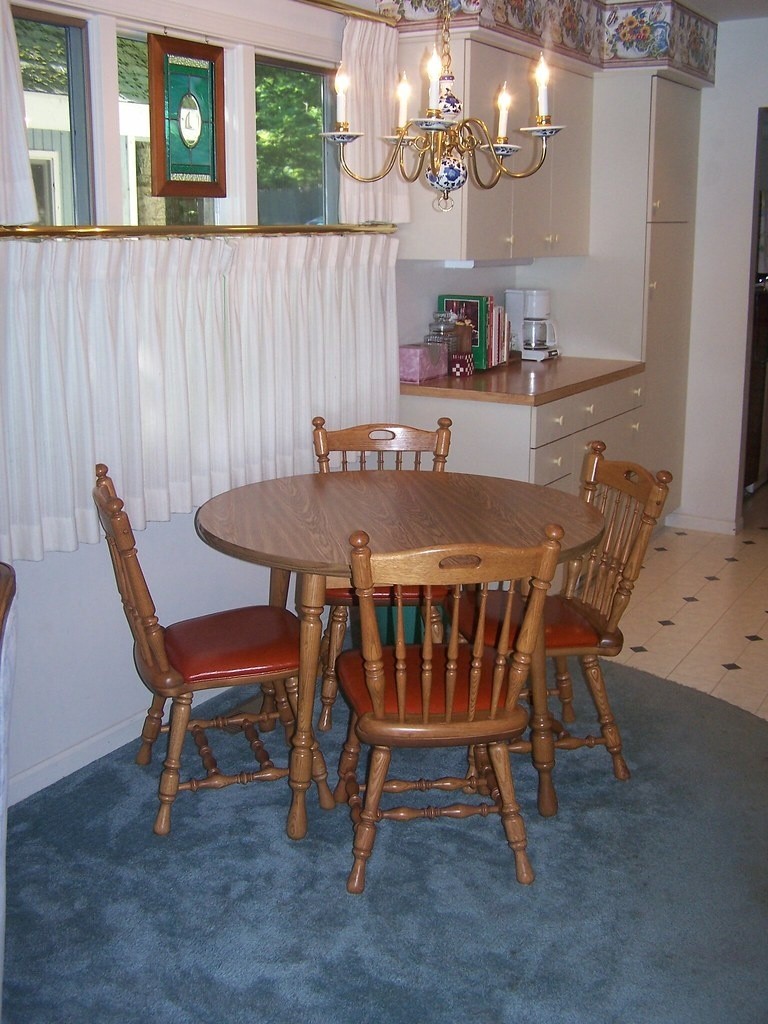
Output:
[319,2,564,212]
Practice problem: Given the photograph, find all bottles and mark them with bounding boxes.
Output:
[424,311,459,363]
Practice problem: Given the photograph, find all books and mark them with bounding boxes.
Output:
[438,294,511,370]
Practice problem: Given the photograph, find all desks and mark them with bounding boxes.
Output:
[196,469,607,840]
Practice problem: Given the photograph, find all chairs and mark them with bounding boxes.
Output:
[332,524,565,893]
[313,417,453,729]
[94,464,336,836]
[443,441,673,817]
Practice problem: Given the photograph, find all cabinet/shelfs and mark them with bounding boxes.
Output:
[554,77,701,513]
[399,357,645,592]
[398,33,592,260]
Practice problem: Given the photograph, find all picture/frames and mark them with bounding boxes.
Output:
[147,33,226,199]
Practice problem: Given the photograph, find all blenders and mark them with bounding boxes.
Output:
[521,289,559,362]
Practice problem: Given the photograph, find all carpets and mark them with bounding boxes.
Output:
[1,629,768,1024]
